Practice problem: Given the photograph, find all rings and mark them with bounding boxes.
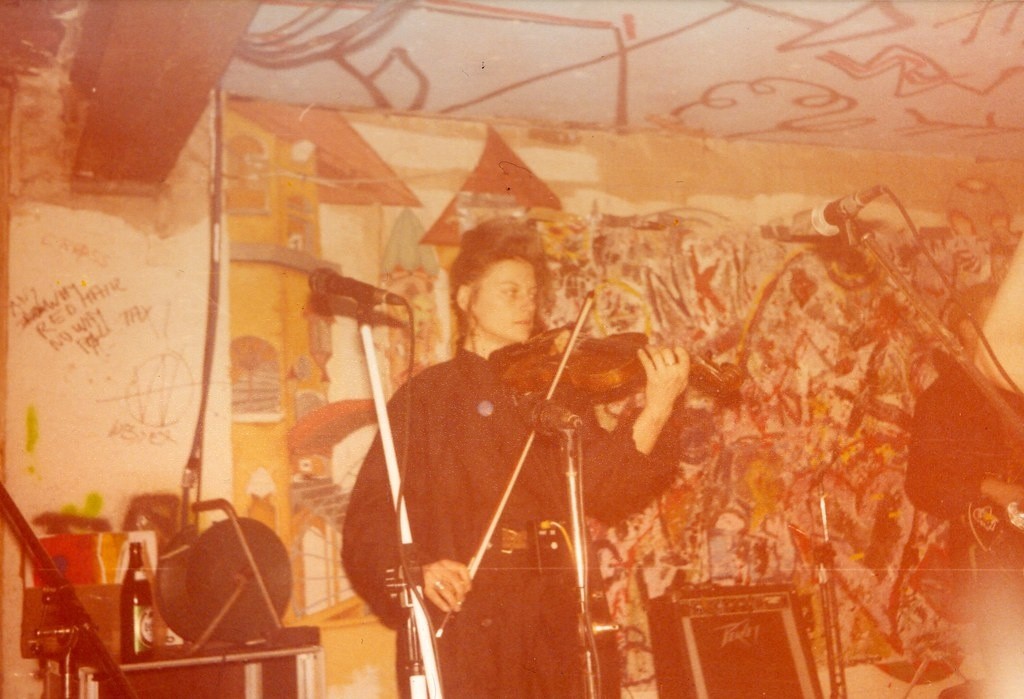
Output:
[433,581,446,592]
[667,361,675,368]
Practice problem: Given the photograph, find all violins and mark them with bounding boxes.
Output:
[502,327,743,398]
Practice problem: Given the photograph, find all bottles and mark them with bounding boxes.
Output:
[120,542,156,662]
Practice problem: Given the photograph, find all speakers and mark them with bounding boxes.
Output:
[647,582,824,699]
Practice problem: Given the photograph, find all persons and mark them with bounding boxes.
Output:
[901,282,1024,699]
[341,216,692,699]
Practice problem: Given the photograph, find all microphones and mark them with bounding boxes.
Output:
[311,267,408,308]
[532,400,582,436]
[812,185,886,236]
